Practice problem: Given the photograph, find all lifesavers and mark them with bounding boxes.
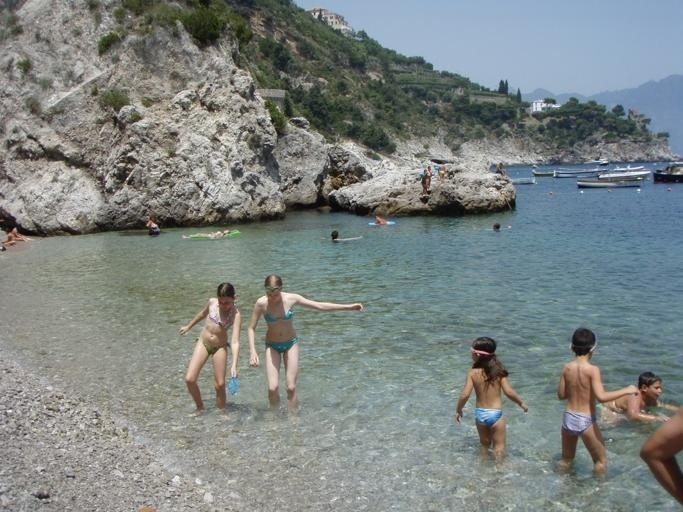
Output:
[190,230,241,240]
[369,220,397,225]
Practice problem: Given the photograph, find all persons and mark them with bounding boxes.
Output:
[146,215,160,235]
[557,328,641,485]
[455,336,530,466]
[182,229,231,240]
[485,159,506,177]
[420,162,452,195]
[639,405,683,506]
[491,223,501,233]
[178,282,241,418]
[247,275,365,413]
[322,231,363,244]
[596,371,679,423]
[374,215,386,226]
[0,226,35,254]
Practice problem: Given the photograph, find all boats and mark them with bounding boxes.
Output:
[511,158,683,188]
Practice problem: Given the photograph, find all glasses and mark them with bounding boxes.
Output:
[265,287,280,293]
